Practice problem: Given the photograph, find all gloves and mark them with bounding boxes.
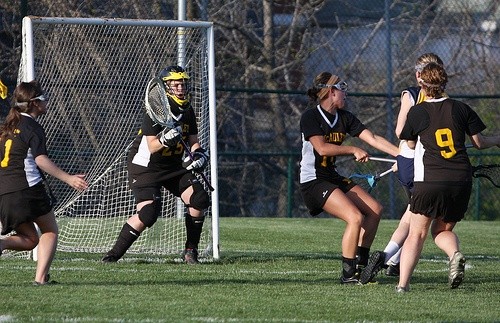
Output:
[182,148,209,173]
[157,125,185,148]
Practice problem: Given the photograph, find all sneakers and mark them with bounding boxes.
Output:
[447,251,466,289]
[394,283,409,292]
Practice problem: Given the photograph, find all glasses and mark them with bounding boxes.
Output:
[318,81,348,92]
[14,94,46,106]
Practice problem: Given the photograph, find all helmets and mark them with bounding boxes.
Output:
[160,65,191,112]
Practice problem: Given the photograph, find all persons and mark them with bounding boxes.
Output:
[0,81,89,286]
[102,66,209,264]
[300,72,399,286]
[396,62,500,293]
[360,53,450,283]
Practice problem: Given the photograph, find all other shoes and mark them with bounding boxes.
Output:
[385,264,400,276]
[180,248,200,264]
[31,280,57,285]
[358,250,385,284]
[339,273,379,285]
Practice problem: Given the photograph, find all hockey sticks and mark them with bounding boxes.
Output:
[348,145,474,194]
[144,77,215,192]
[367,156,500,188]
